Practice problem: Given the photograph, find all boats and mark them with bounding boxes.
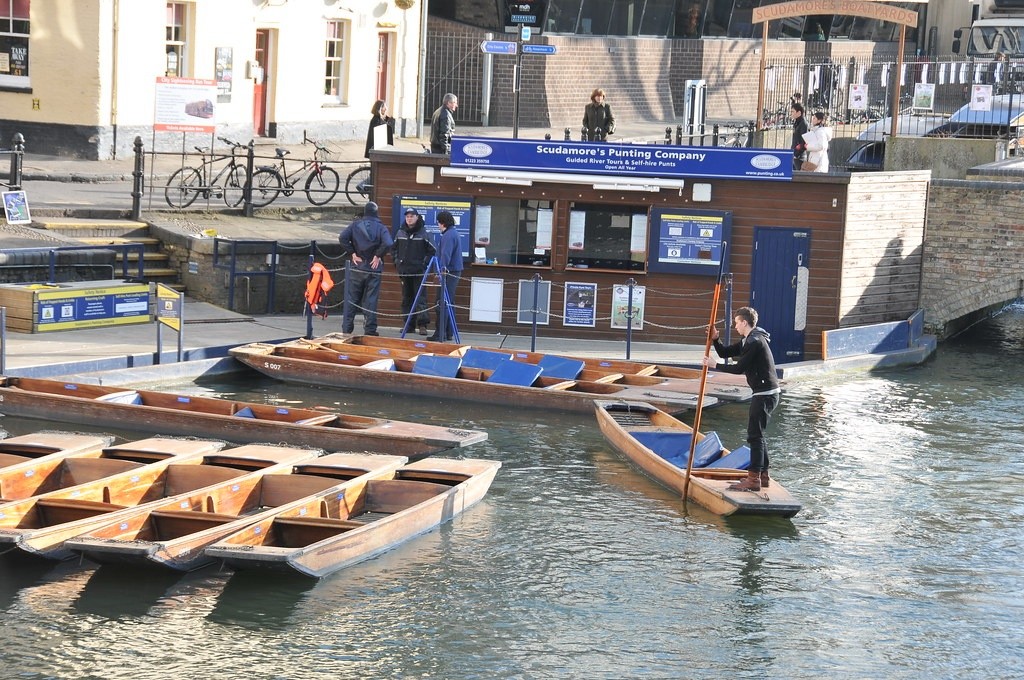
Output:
[591,398,805,518]
[228,341,720,415]
[0,430,123,471]
[0,436,228,509]
[0,443,324,561]
[0,376,492,458]
[306,332,758,401]
[206,457,507,577]
[61,454,409,575]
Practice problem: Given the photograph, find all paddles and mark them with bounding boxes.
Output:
[680,239,729,520]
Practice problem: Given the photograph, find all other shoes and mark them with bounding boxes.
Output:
[446,334,453,339]
[363,332,379,336]
[400,326,415,333]
[419,327,426,334]
[426,330,446,341]
[355,186,366,199]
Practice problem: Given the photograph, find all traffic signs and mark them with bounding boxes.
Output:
[479,41,517,55]
[522,44,556,55]
[504,26,519,33]
[510,14,538,24]
[530,26,543,36]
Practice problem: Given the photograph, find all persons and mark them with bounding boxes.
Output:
[393,206,437,335]
[430,94,459,155]
[340,202,393,337]
[704,306,780,494]
[986,52,1005,94]
[791,103,810,170]
[802,112,833,174]
[355,98,393,199]
[427,211,464,340]
[581,88,617,140]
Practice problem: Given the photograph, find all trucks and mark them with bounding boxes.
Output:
[950,0,1024,81]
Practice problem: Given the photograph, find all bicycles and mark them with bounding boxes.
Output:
[344,165,373,206]
[164,136,251,209]
[715,64,911,148]
[243,130,344,208]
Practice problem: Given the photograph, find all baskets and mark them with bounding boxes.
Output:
[315,139,343,161]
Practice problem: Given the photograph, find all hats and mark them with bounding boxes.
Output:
[403,207,419,215]
[364,202,378,216]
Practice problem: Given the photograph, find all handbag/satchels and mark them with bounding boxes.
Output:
[800,161,818,172]
[604,105,616,134]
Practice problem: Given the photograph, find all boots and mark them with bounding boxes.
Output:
[740,471,769,487]
[729,471,760,491]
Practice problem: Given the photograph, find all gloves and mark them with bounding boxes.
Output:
[804,144,807,149]
[795,144,801,150]
